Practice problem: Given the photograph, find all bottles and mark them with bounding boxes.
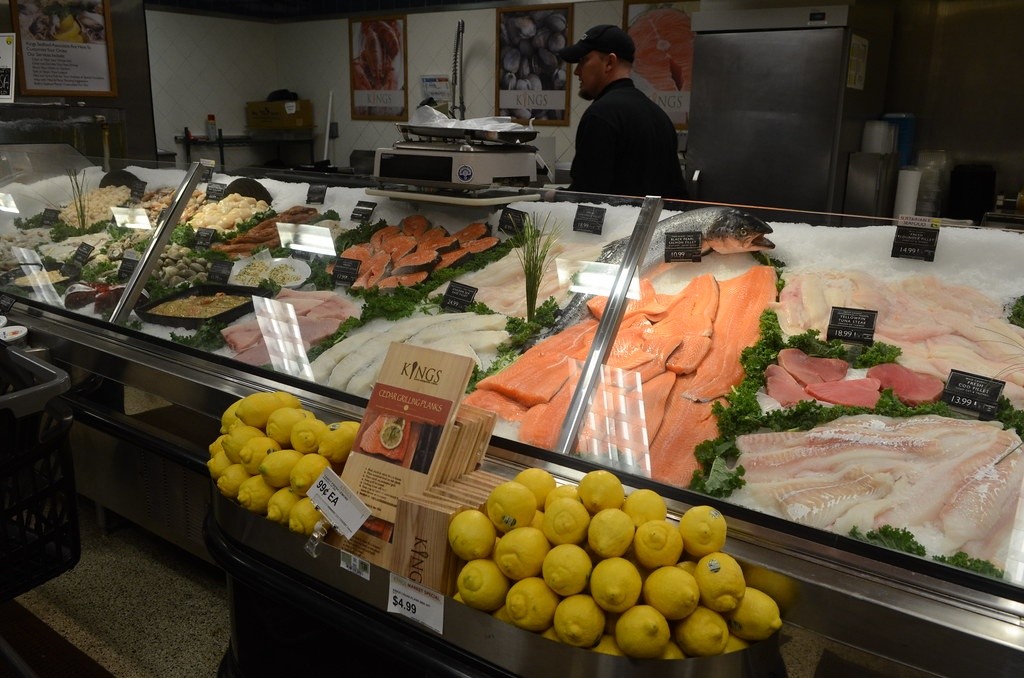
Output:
[207,114,217,141]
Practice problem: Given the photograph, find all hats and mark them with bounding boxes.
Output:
[559,25,635,63]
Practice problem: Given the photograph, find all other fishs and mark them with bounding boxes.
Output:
[518,205,776,355]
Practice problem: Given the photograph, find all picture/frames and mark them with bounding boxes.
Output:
[348,14,408,121]
[494,4,573,126]
[9,0,118,96]
[622,0,699,131]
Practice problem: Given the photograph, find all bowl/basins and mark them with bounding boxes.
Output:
[0,262,82,296]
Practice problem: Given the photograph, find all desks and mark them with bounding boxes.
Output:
[175,129,314,166]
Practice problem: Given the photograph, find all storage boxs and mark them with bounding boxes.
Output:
[247,99,314,128]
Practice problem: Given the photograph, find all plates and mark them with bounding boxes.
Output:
[229,257,312,290]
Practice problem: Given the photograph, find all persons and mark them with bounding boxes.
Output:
[542,24,685,203]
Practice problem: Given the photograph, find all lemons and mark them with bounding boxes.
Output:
[205,391,361,539]
[448,466,782,659]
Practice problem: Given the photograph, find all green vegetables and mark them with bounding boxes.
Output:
[13,204,564,394]
[686,255,1024,582]
